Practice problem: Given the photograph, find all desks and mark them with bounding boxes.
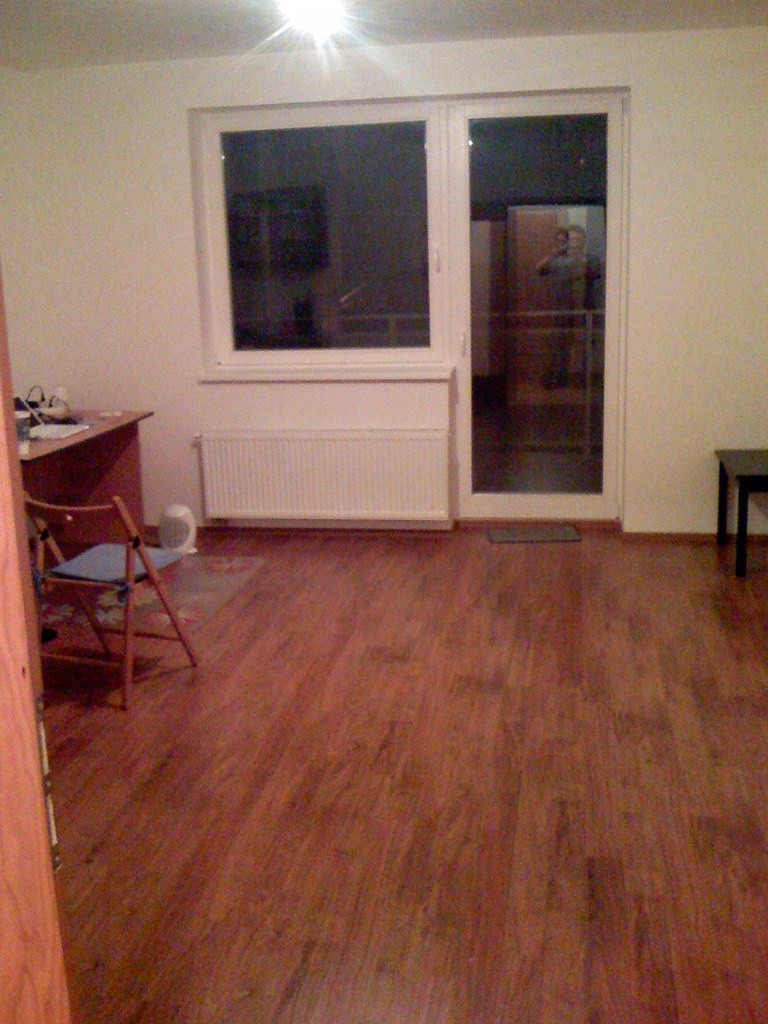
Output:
[16,409,154,548]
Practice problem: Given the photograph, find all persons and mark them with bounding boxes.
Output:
[536,224,605,388]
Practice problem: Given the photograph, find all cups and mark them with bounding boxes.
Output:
[15,411,31,455]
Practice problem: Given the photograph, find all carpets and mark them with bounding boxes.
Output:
[486,524,582,544]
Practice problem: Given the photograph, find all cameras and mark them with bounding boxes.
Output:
[559,248,568,254]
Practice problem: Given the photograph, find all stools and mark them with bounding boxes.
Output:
[714,448,768,578]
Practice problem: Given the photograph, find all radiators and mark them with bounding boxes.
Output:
[194,430,451,524]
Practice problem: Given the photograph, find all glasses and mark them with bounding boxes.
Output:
[21,385,69,408]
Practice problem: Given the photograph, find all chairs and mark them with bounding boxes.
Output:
[23,492,201,711]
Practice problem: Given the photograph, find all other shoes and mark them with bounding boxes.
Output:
[572,371,586,390]
[543,371,571,389]
[39,628,58,644]
